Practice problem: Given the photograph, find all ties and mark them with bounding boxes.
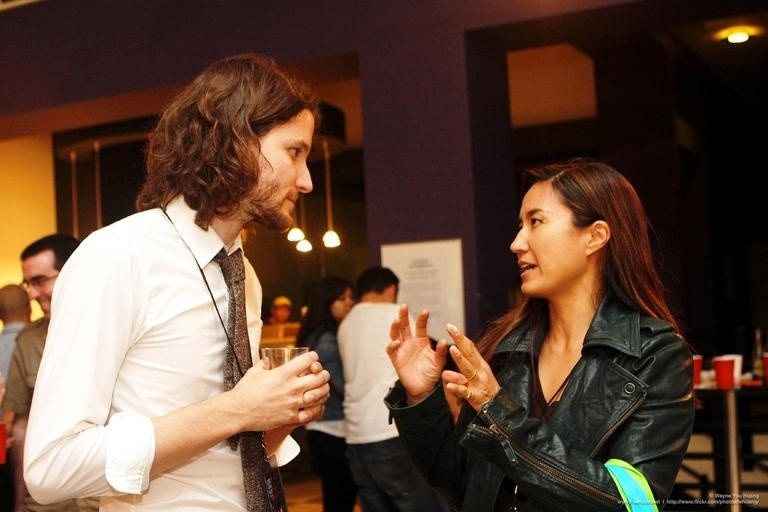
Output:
[212,247,271,512]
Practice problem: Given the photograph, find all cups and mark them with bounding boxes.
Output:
[692,354,703,388]
[711,353,743,388]
[262,346,308,372]
[759,352,768,379]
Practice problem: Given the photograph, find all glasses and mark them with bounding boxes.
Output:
[22,275,57,288]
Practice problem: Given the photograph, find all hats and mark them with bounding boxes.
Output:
[273,295,292,307]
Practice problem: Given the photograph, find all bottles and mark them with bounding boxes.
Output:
[752,330,761,380]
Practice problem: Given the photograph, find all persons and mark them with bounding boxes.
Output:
[0,233,101,512]
[337,266,452,512]
[23,54,331,512]
[294,276,359,512]
[383,157,703,512]
[0,285,32,382]
[262,295,293,324]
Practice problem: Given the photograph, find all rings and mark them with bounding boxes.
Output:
[465,390,472,399]
[467,369,477,382]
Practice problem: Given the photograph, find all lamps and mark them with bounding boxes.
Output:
[322,142,341,248]
[295,192,313,253]
[287,211,305,242]
